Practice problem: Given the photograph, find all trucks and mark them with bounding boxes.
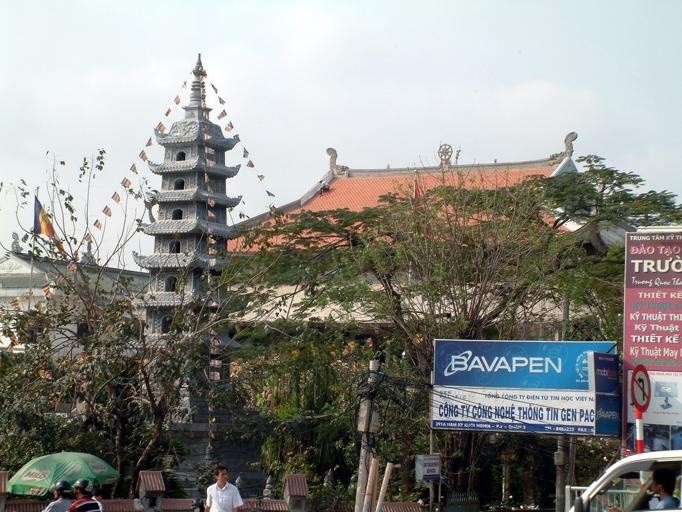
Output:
[568,449,682,512]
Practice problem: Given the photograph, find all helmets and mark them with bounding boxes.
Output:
[73,478,93,493]
[54,480,72,493]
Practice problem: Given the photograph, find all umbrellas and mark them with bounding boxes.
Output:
[7,448,121,498]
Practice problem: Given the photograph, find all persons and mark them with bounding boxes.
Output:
[65,478,101,512]
[91,488,105,512]
[41,480,74,512]
[204,465,244,512]
[608,468,680,512]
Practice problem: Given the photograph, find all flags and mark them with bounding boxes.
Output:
[33,196,67,258]
